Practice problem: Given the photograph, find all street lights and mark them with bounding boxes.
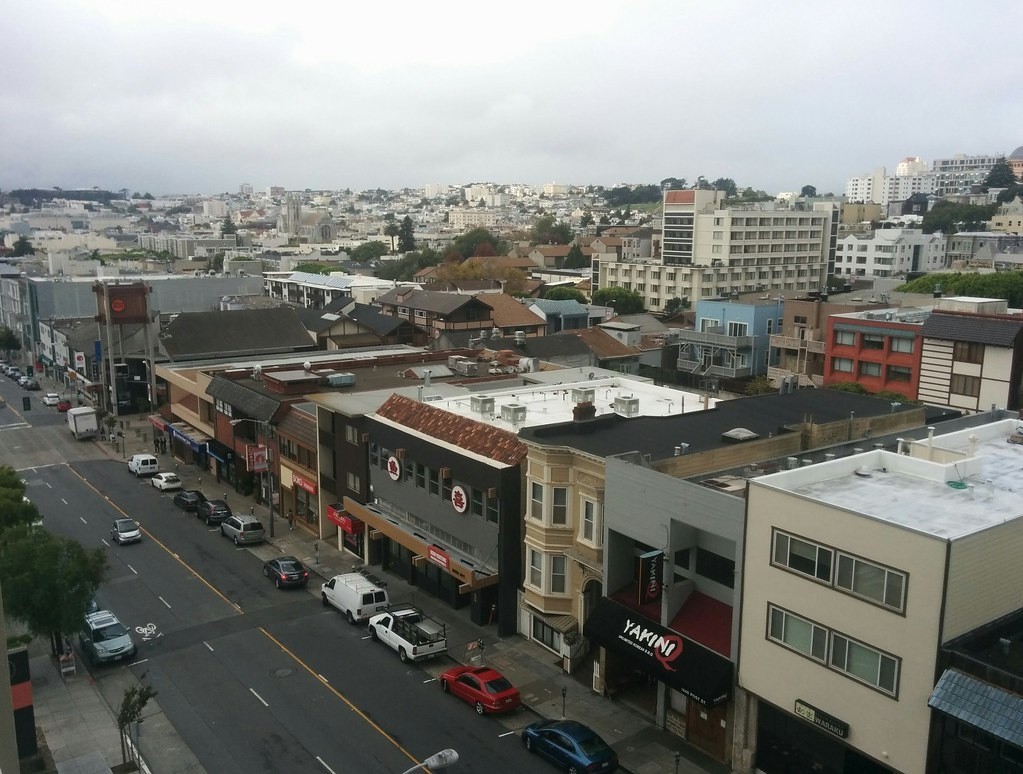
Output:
[229,418,275,539]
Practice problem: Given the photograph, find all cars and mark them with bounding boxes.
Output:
[111,518,142,545]
[42,393,60,406]
[521,718,619,774]
[263,555,309,590]
[151,472,182,492]
[440,665,521,716]
[77,597,139,668]
[0,359,40,391]
[173,490,209,511]
[57,399,72,412]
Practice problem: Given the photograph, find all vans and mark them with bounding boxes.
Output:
[220,513,266,547]
[127,454,160,477]
[320,571,393,625]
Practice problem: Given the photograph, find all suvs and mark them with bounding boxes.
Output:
[196,499,233,526]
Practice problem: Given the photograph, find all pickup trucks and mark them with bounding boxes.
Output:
[367,602,448,665]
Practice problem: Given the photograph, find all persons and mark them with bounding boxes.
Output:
[153,437,167,453]
[100,426,106,441]
[109,432,115,442]
[286,508,294,534]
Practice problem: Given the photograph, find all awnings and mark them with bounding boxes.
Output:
[583,596,736,708]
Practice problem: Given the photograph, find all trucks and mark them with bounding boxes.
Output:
[65,406,99,440]
